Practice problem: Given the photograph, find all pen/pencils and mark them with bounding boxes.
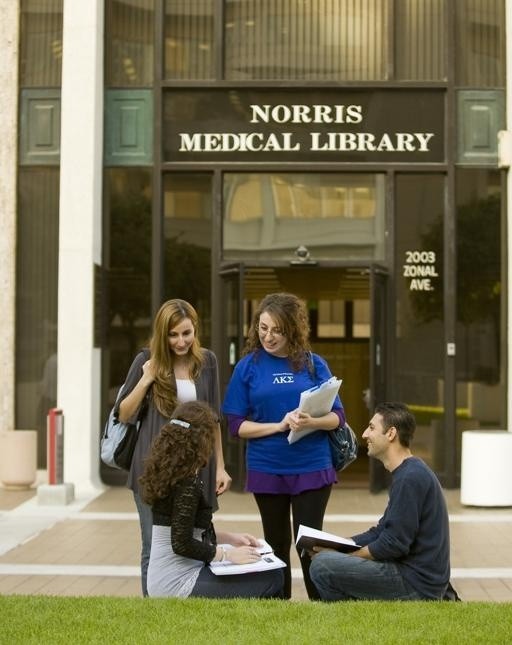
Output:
[260,552,271,555]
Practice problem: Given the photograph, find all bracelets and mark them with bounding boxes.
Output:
[219,547,226,561]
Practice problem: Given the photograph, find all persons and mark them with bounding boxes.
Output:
[217,291,347,598]
[132,400,289,599]
[307,400,450,601]
[111,299,235,599]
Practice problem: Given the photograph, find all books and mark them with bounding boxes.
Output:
[207,537,288,579]
[295,523,363,558]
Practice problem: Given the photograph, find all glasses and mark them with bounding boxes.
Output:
[255,324,287,338]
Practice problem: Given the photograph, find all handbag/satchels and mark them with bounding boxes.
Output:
[99,349,150,472]
[331,421,358,472]
